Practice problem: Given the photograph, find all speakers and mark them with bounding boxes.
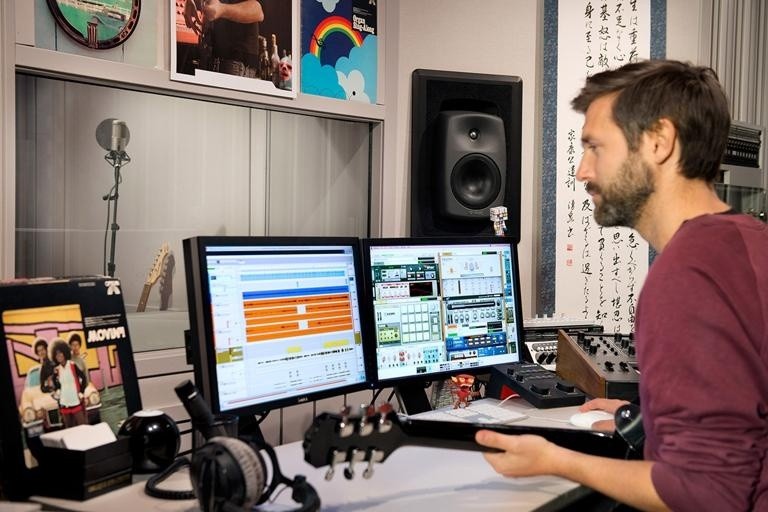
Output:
[411,69,523,244]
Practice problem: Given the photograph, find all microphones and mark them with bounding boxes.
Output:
[111,119,126,167]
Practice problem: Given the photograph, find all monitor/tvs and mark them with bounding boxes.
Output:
[360,236,526,390]
[182,235,374,418]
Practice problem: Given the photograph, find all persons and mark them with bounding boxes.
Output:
[68,333,87,380]
[181,0,266,80]
[472,57,767,512]
[16,339,59,422]
[40,341,88,430]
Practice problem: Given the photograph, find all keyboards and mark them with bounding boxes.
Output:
[414,403,529,426]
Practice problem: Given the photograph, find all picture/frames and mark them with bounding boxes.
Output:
[168,0,298,99]
[47,0,142,49]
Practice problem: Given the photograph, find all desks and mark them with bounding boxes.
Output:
[30,386,688,511]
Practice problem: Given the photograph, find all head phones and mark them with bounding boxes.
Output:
[190,435,320,512]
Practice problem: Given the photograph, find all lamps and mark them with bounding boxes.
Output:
[93,117,131,282]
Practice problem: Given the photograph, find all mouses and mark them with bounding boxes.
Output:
[570,409,616,430]
